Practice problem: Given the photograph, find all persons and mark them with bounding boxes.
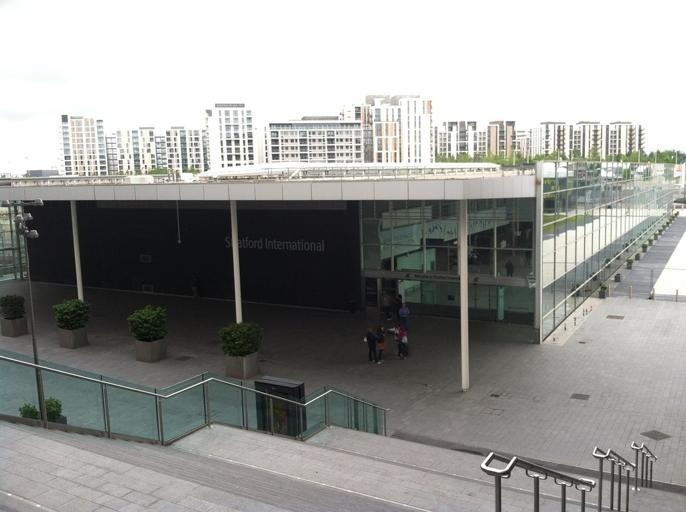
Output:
[365,290,410,364]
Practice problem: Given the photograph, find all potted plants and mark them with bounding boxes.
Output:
[221,322,262,380]
[598,211,680,298]
[20,402,41,420]
[52,299,91,349]
[126,304,167,362]
[44,397,67,424]
[0,294,27,337]
